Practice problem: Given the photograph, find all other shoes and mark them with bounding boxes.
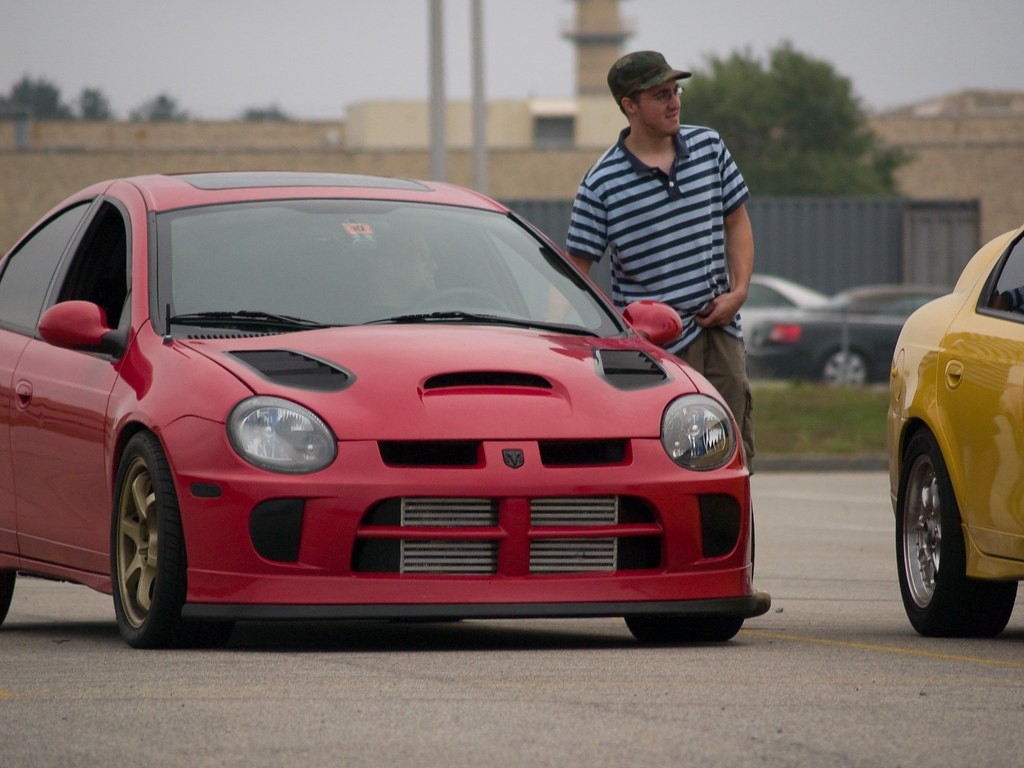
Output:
[745,592,771,618]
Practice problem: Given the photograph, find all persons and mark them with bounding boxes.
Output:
[540,51,771,621]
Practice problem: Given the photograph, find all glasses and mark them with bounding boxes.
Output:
[647,86,684,105]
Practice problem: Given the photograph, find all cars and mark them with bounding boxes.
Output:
[1,171,772,650]
[884,224,1024,640]
[744,285,950,390]
[725,275,831,348]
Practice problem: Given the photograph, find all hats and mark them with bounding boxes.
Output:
[607,51,691,102]
